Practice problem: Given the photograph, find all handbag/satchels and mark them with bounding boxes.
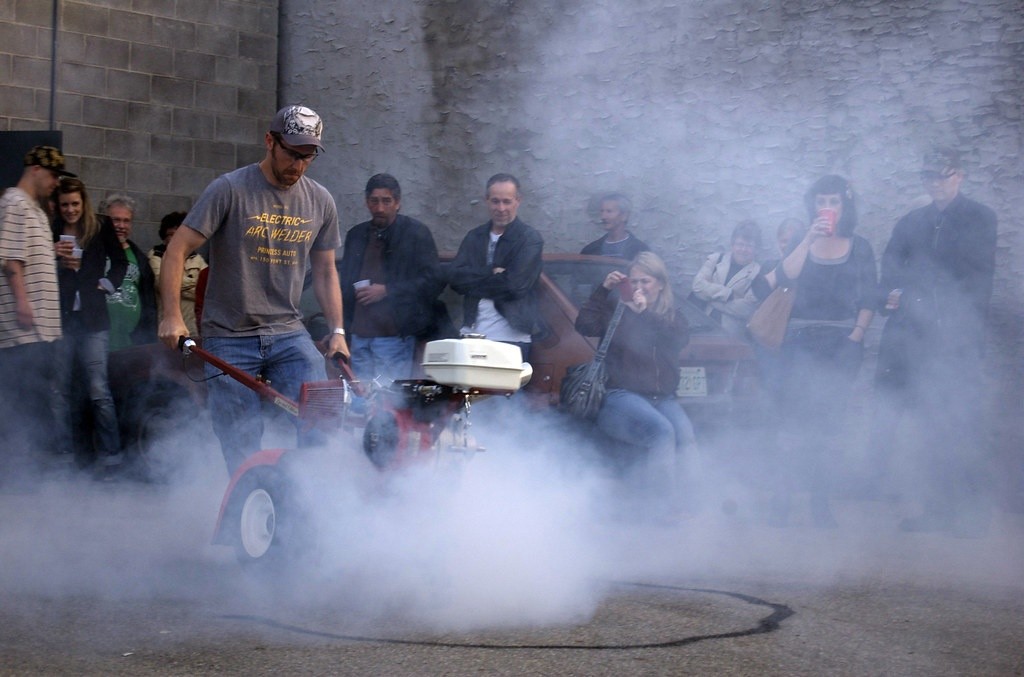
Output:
[558,361,608,422]
[687,253,725,311]
[746,279,798,349]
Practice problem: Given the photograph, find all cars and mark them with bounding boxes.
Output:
[108,252,764,492]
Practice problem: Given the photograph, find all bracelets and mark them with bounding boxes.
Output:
[329,327,346,337]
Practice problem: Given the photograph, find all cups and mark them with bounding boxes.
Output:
[59,235,76,240]
[353,279,370,289]
[817,208,837,236]
[72,249,83,258]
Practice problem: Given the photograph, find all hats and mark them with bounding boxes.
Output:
[26,145,77,177]
[270,106,326,153]
[921,148,958,175]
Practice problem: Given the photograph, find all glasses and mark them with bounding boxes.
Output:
[921,168,955,184]
[274,134,318,162]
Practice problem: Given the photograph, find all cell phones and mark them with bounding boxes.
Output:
[619,277,633,302]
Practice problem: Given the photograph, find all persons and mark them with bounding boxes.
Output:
[575,150,1004,519]
[158,105,351,481]
[0,145,209,496]
[337,173,446,416]
[448,174,544,362]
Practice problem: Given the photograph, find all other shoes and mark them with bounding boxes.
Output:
[104,462,121,483]
